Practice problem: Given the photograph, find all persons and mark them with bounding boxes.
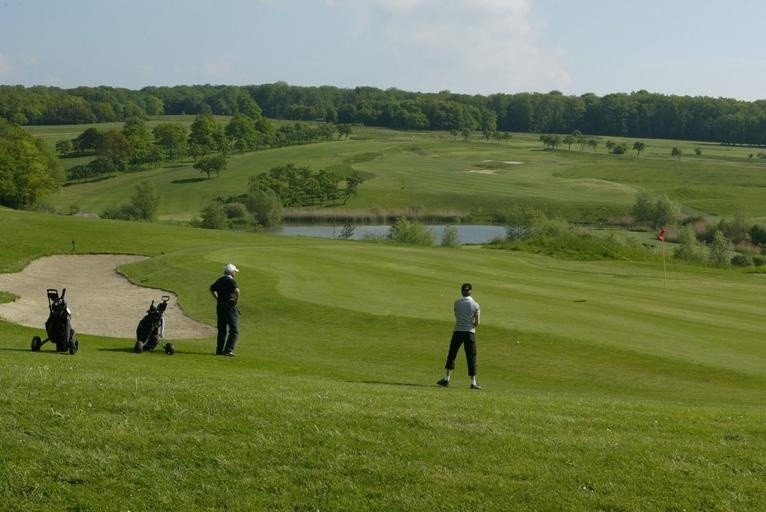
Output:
[209,264,240,357]
[437,283,482,389]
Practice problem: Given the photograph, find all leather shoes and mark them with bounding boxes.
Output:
[437,378,450,387]
[471,383,482,389]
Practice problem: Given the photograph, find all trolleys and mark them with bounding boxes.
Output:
[131,295,176,355]
[27,288,80,356]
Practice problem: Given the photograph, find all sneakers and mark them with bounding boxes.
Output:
[215,351,236,357]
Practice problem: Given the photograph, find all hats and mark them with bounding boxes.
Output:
[225,264,240,273]
[461,283,473,291]
